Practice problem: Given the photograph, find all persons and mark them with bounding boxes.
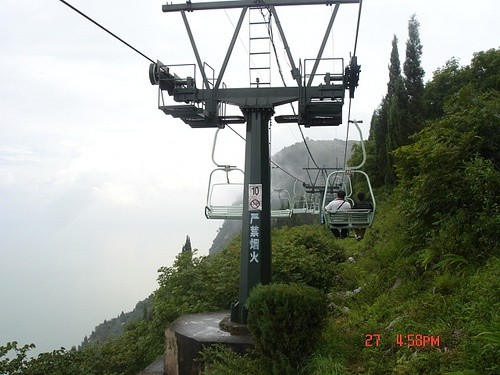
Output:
[325,190,352,239]
[350,192,374,242]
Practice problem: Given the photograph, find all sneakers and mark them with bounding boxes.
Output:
[355,234,360,241]
[356,235,363,240]
